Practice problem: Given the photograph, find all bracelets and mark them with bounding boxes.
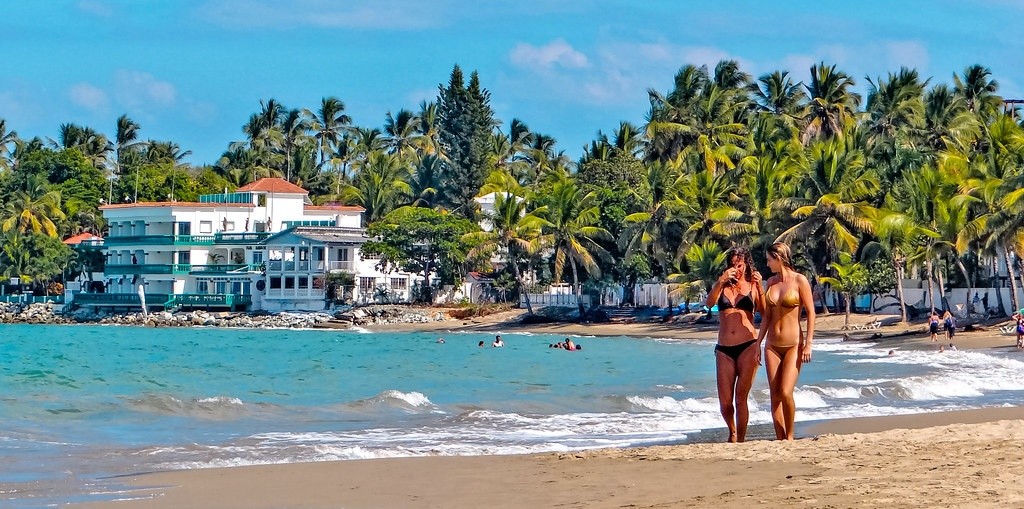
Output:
[759,291,765,295]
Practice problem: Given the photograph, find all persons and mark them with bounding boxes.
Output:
[479,341,484,347]
[549,338,581,350]
[929,311,940,341]
[946,314,956,339]
[943,311,952,340]
[754,242,815,440]
[492,335,504,347]
[940,343,958,353]
[1012,313,1024,348]
[706,249,767,442]
[973,293,988,312]
